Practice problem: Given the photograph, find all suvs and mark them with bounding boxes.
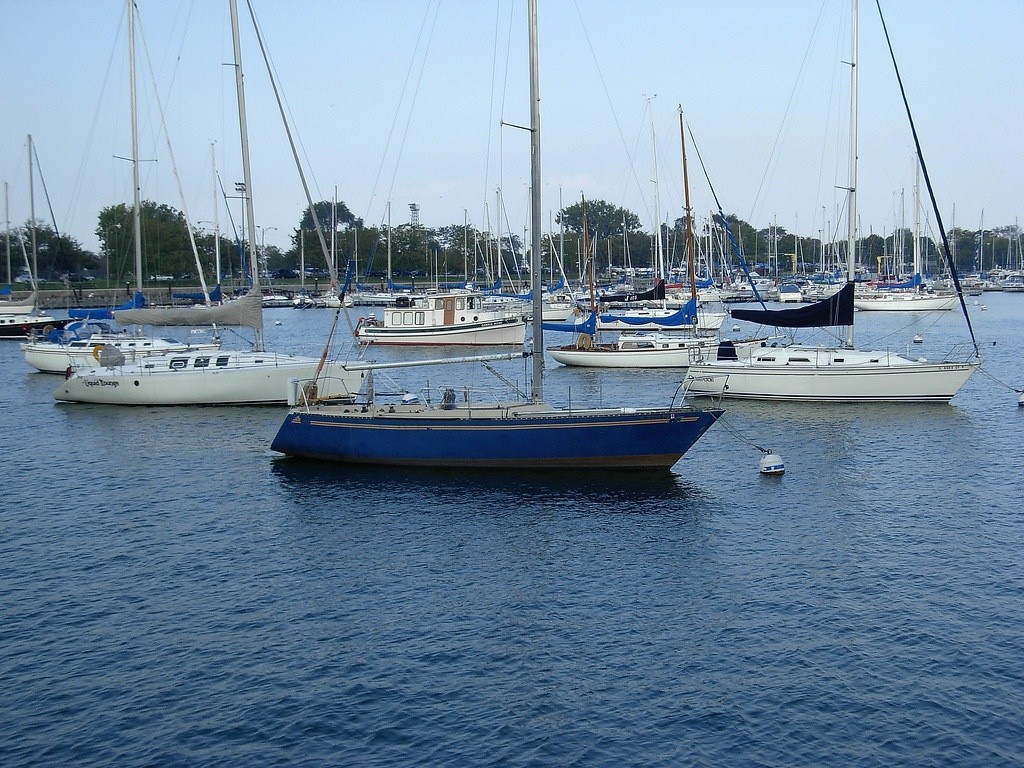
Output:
[272,269,300,279]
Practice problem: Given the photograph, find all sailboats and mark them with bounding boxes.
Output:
[51,0,370,406]
[514,176,1023,308]
[201,141,512,307]
[268,0,728,473]
[0,134,82,338]
[853,151,968,311]
[574,95,725,330]
[678,0,982,404]
[546,102,788,369]
[0,181,38,315]
[20,0,224,374]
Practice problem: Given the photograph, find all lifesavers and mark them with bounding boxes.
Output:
[578,333,591,350]
[92,345,105,363]
[299,380,319,406]
[42,325,54,340]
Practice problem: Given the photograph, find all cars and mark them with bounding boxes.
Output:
[335,268,353,278]
[59,273,90,282]
[151,275,173,281]
[392,269,426,277]
[365,270,386,277]
[14,275,46,284]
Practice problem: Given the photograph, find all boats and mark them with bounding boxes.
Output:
[356,292,527,346]
[68,291,145,319]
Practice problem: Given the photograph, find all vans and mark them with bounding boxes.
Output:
[306,268,324,278]
